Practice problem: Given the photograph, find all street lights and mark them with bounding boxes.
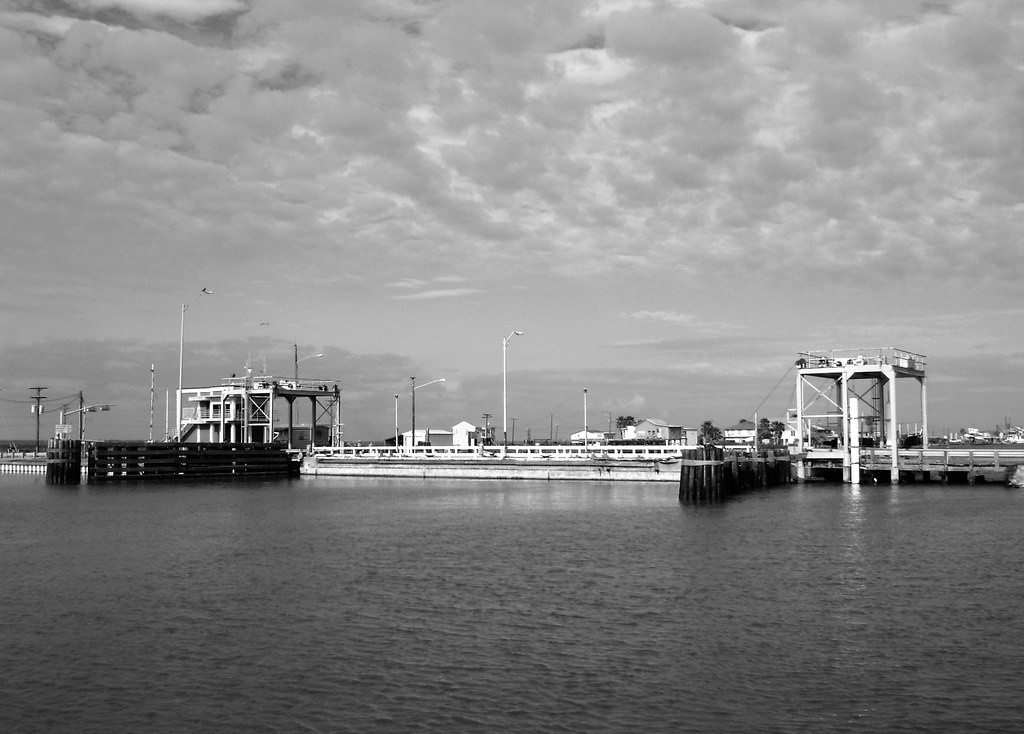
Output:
[394,394,398,452]
[584,388,588,452]
[409,375,446,455]
[499,330,524,458]
[177,287,214,442]
[293,343,325,424]
